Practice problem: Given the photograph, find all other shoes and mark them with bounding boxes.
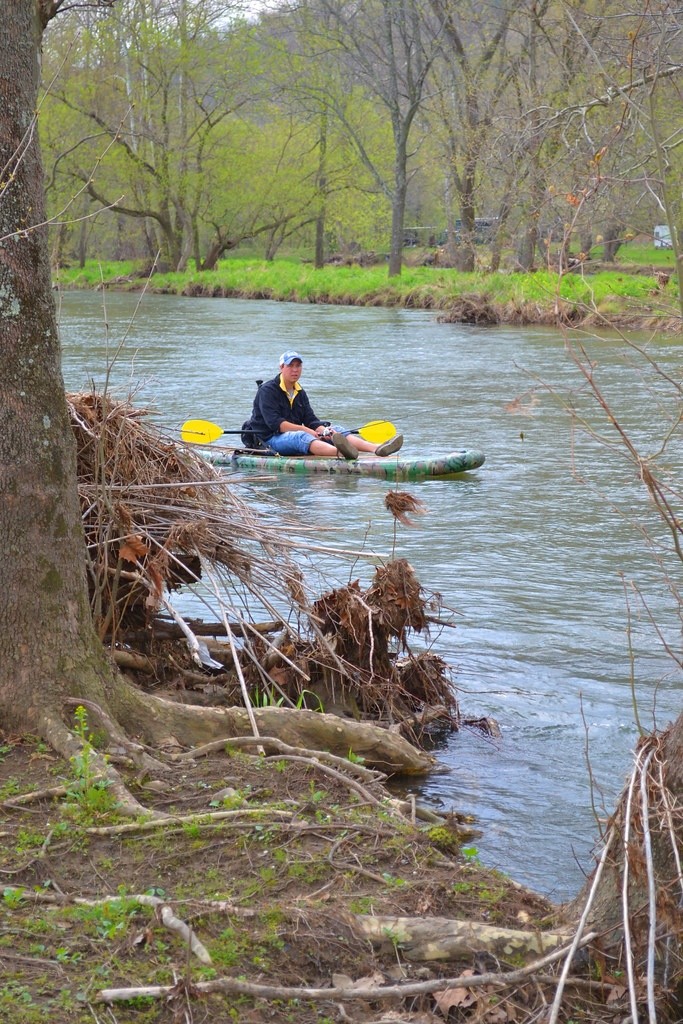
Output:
[333,434,358,459]
[375,432,403,456]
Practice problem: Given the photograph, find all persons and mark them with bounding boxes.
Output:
[250,350,404,459]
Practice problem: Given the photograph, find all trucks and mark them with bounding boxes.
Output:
[653,226,679,250]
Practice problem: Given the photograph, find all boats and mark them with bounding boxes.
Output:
[174,438,486,479]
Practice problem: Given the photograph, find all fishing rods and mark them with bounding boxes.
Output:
[317,401,473,440]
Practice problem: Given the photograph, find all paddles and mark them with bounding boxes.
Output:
[180,418,398,446]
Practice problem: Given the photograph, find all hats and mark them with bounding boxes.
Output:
[280,350,303,366]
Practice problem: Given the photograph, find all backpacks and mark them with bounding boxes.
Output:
[241,420,268,451]
